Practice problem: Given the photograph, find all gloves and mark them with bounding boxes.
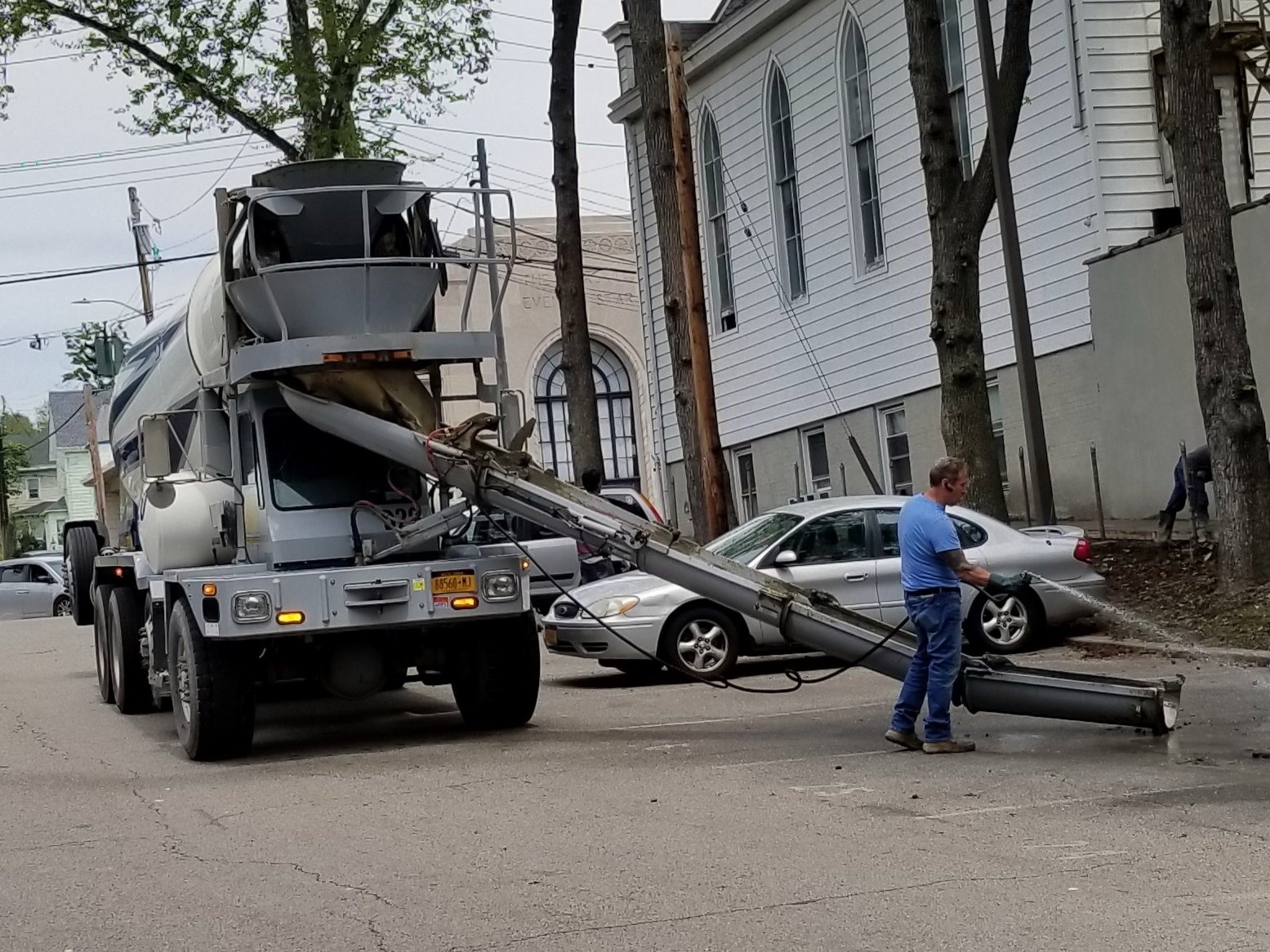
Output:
[983,570,1032,594]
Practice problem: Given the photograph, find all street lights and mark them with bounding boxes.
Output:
[71,186,163,328]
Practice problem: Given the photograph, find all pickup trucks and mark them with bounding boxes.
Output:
[459,501,581,603]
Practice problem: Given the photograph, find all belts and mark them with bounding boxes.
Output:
[902,587,961,596]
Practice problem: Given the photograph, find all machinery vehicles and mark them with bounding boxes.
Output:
[60,156,1185,766]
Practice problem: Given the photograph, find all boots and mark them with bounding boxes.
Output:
[1189,509,1219,543]
[1155,510,1178,546]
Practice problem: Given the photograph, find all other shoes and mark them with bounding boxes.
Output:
[923,738,975,753]
[885,727,924,750]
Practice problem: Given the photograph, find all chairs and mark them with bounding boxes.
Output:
[798,526,846,564]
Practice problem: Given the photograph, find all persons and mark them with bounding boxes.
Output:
[885,455,1033,755]
[575,466,618,585]
[1151,443,1219,551]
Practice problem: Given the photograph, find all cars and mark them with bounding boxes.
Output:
[0,553,73,624]
[19,548,62,559]
[539,494,1108,682]
[572,485,667,581]
